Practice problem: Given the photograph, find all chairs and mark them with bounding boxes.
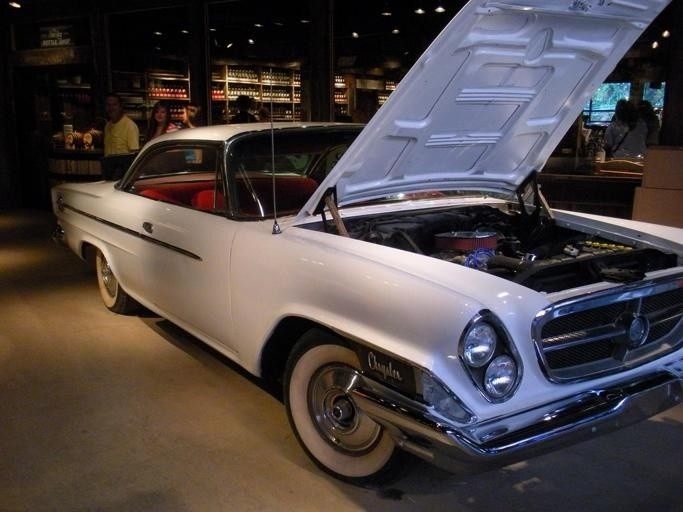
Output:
[135,175,319,217]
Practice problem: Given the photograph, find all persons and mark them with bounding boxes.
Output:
[603,100,662,158]
[101,93,140,181]
[146,92,378,142]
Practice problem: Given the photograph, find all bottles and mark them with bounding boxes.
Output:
[147,68,347,119]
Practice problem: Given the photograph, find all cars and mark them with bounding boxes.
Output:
[51,1,683,485]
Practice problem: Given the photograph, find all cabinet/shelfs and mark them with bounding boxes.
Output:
[110,59,401,146]
